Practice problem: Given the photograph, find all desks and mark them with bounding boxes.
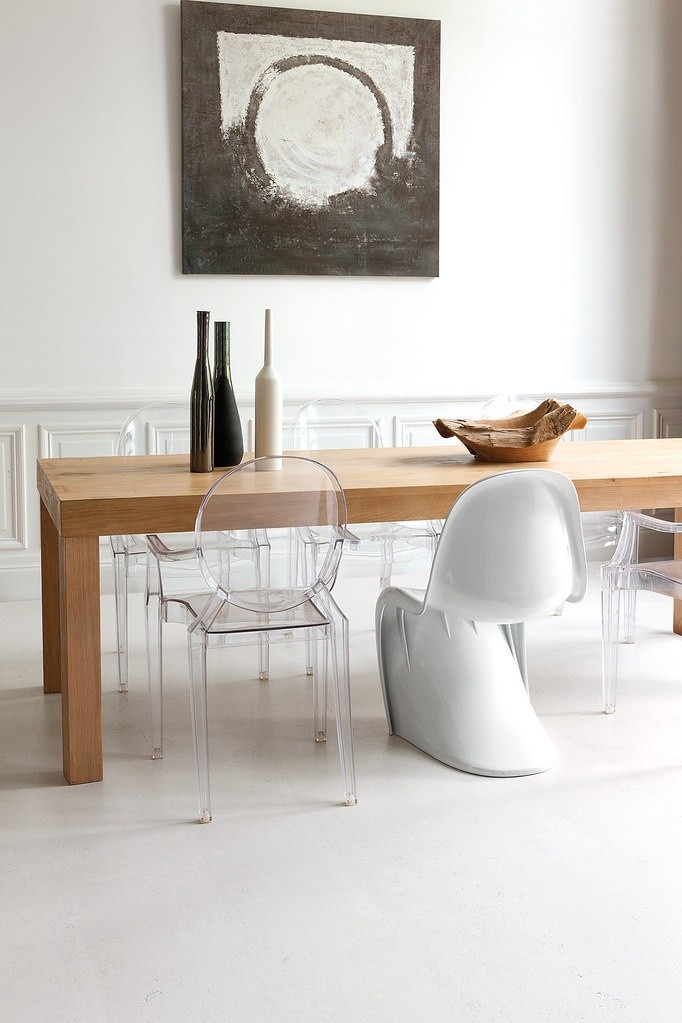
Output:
[35,437,682,785]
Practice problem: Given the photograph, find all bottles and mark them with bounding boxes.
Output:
[189,312,214,472]
[255,309,282,472]
[212,321,244,469]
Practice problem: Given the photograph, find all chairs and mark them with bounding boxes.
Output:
[104,389,682,827]
